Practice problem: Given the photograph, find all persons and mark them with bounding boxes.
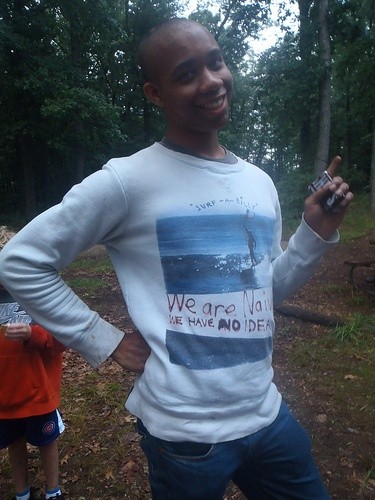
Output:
[2,226,69,500]
[1,18,355,499]
